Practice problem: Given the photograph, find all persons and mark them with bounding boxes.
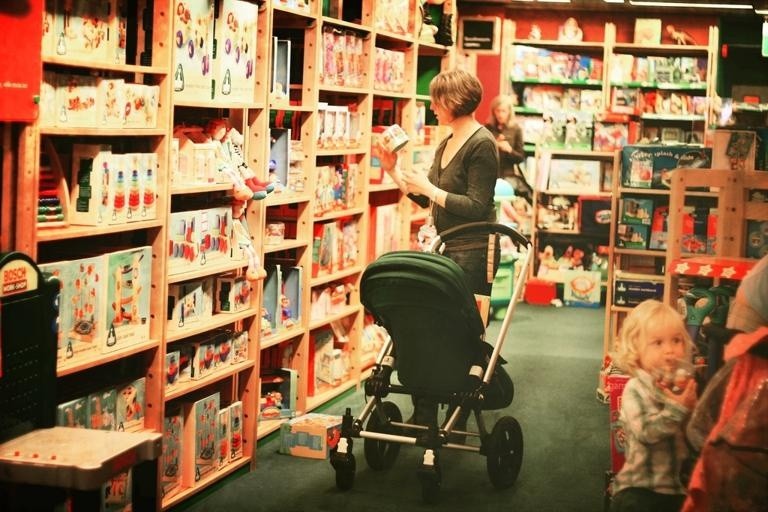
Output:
[205,118,274,281]
[610,298,697,511]
[484,94,532,231]
[376,70,500,445]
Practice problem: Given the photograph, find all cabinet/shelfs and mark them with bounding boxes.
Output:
[18,0,455,508]
[495,17,765,347]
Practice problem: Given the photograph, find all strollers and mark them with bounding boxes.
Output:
[327,221,534,494]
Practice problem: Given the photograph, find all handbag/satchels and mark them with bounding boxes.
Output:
[501,160,533,205]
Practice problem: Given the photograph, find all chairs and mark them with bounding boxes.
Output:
[0,252,167,508]
